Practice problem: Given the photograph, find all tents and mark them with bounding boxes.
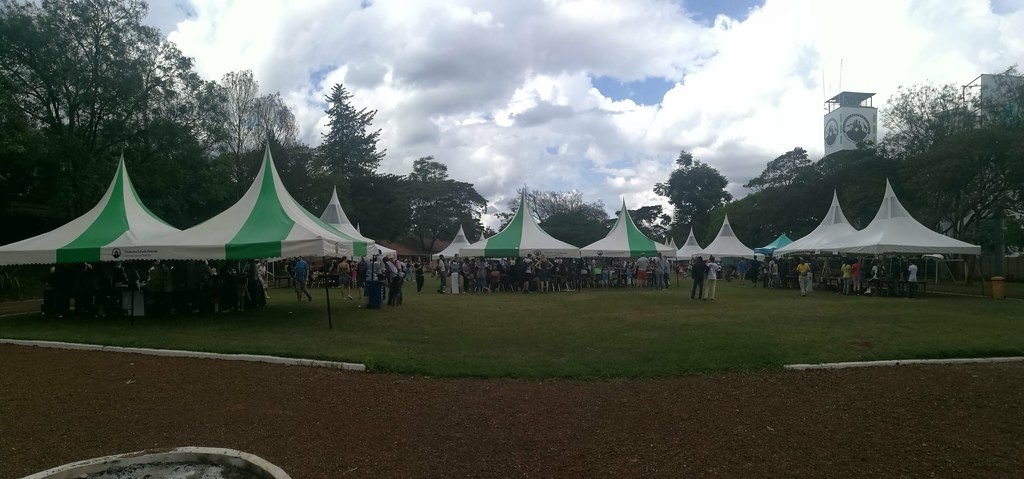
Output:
[431,178,986,295]
[154,138,377,328]
[318,186,397,281]
[0,138,186,329]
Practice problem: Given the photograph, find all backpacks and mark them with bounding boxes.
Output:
[874,264,884,279]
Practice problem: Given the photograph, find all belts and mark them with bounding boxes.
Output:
[801,274,807,276]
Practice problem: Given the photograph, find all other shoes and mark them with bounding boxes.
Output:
[309,297,312,302]
[358,298,362,300]
[267,297,271,299]
[348,295,353,300]
[802,295,805,296]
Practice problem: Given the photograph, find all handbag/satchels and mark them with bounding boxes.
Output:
[655,265,661,272]
[393,276,402,289]
[522,259,527,271]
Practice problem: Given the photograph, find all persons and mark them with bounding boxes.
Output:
[40,254,919,321]
